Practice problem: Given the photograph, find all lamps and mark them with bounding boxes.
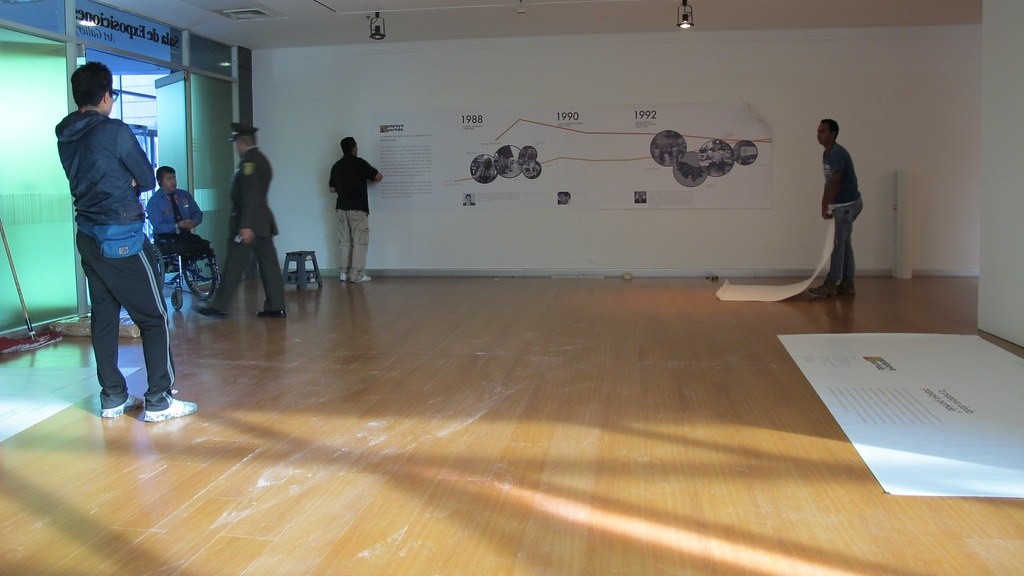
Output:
[369,10,384,38]
[678,1,695,27]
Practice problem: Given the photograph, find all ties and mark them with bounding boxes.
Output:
[167,192,183,223]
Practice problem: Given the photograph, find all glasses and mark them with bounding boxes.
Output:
[107,90,120,102]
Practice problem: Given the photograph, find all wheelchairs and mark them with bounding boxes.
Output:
[148,232,221,310]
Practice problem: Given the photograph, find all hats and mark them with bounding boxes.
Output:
[228,123,260,142]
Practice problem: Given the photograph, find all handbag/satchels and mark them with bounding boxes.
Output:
[81,222,146,259]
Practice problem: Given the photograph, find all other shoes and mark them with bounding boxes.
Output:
[256,309,287,318]
[143,398,199,422]
[339,272,372,283]
[196,306,230,317]
[100,395,142,417]
[808,281,855,297]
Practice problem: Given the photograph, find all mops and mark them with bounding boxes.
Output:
[0,216,65,354]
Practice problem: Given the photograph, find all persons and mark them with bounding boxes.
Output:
[145,165,212,268]
[808,118,864,298]
[463,193,475,206]
[328,137,383,283]
[636,192,646,203]
[54,59,202,424]
[194,123,287,318]
[558,192,571,205]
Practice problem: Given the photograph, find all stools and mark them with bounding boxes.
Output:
[281,249,321,290]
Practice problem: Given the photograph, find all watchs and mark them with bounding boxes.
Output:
[176,224,182,234]
